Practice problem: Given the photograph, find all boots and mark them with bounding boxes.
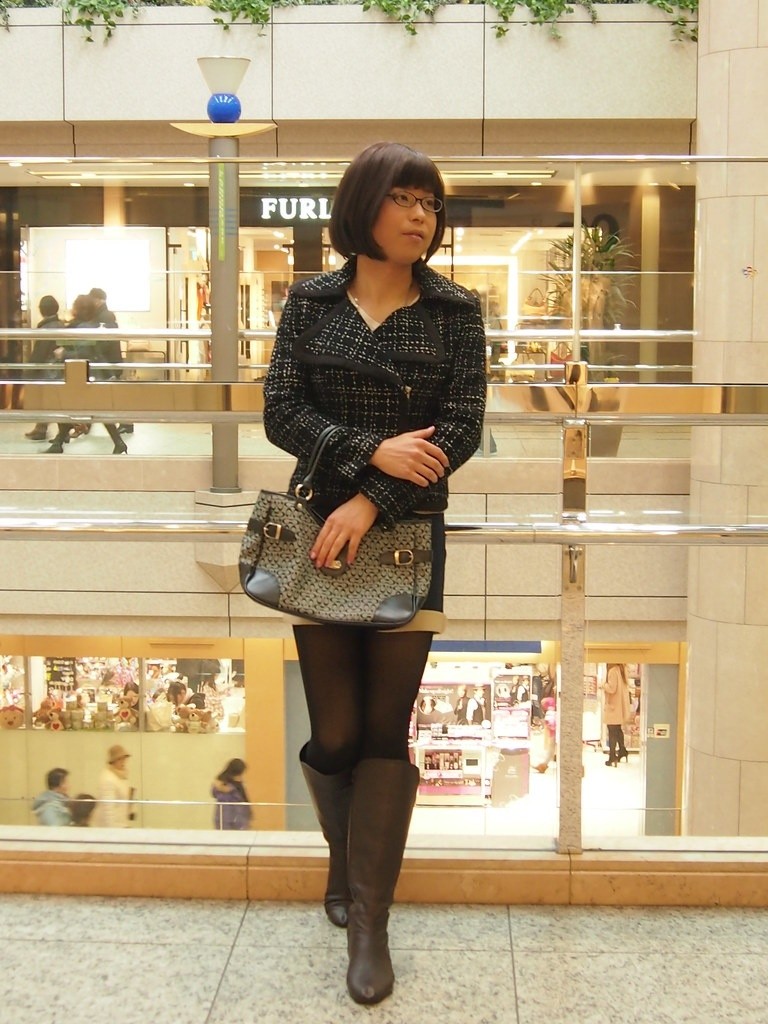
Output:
[297,742,349,929]
[348,757,420,1003]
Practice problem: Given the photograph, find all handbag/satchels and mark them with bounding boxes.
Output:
[237,428,432,627]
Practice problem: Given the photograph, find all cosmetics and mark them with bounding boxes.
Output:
[423,751,460,770]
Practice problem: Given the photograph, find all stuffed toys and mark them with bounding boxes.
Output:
[170,707,220,732]
[0,695,65,731]
[112,696,141,726]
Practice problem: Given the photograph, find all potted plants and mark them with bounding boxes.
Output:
[540,220,643,383]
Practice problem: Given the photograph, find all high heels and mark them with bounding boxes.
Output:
[113,438,128,455]
[606,756,619,768]
[44,444,63,454]
[617,749,628,763]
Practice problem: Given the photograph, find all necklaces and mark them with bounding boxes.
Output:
[351,283,412,310]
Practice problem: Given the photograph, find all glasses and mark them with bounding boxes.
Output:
[386,190,444,213]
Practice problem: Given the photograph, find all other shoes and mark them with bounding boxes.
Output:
[530,762,548,774]
[69,425,84,438]
[26,429,46,441]
[118,422,133,435]
[50,433,71,444]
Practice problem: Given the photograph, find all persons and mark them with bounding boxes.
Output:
[19,287,132,456]
[196,659,224,720]
[167,681,187,705]
[259,141,489,1004]
[599,663,629,768]
[211,757,251,830]
[94,745,139,827]
[125,682,139,710]
[68,794,96,827]
[31,767,74,826]
[531,737,585,778]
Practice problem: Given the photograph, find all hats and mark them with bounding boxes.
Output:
[107,745,130,765]
[90,288,107,302]
